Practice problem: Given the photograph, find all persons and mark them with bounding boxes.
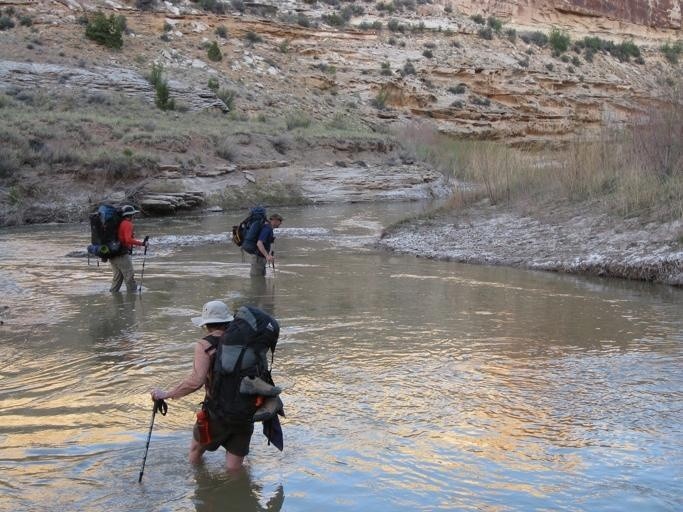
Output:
[248,213,284,275]
[109,206,148,293]
[150,299,258,469]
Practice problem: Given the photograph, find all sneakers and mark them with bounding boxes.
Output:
[239,376,281,396]
[252,396,283,422]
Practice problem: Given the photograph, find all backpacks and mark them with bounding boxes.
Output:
[88,204,128,262]
[232,207,272,255]
[199,305,283,424]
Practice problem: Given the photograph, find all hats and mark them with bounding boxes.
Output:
[121,205,140,217]
[191,301,235,328]
[270,214,283,220]
